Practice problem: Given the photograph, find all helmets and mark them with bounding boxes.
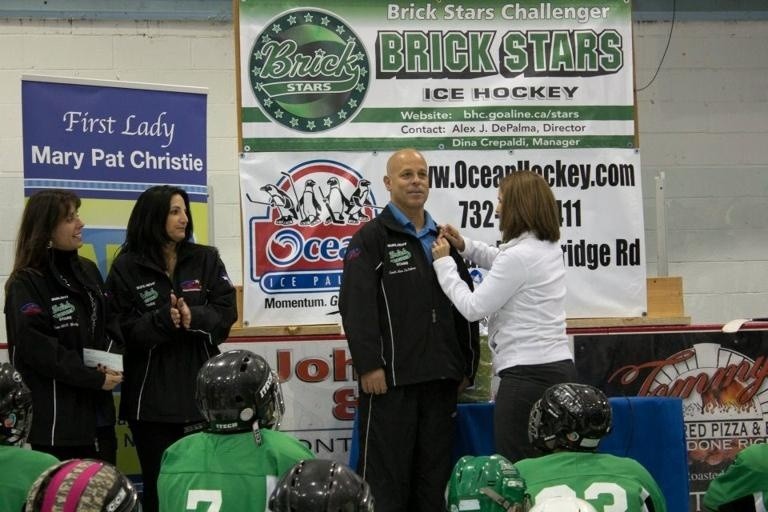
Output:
[527,381,612,451]
[193,349,285,433]
[0,362,33,447]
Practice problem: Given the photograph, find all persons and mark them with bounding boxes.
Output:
[429,170,578,460]
[1,360,64,511]
[701,441,768,512]
[156,348,317,512]
[513,381,667,511]
[335,148,482,510]
[100,185,239,510]
[2,188,126,467]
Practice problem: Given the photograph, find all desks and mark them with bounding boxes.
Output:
[352,394,690,511]
